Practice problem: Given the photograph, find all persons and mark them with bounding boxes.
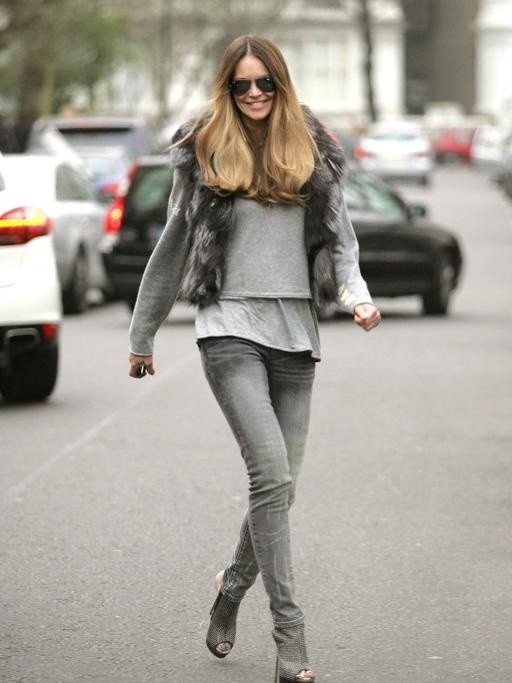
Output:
[127,34,382,683]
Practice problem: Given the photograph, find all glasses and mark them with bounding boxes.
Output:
[230,76,274,96]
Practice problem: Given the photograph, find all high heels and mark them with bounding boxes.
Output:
[272,622,315,683]
[206,593,241,658]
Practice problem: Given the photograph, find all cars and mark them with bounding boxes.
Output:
[98,150,466,314]
[351,115,507,187]
